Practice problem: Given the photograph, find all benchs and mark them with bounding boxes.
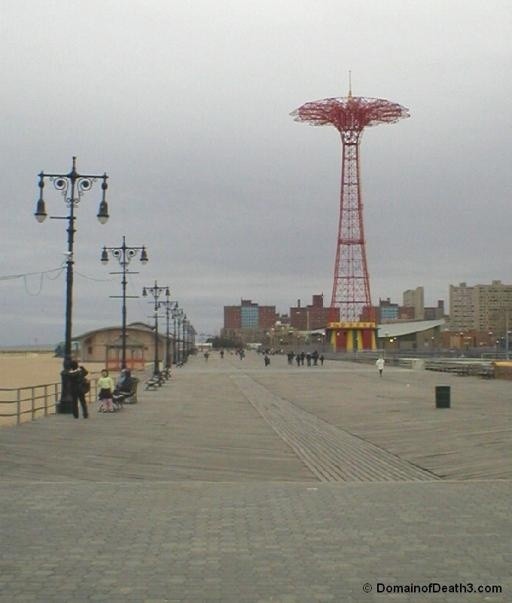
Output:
[455,367,472,376]
[476,367,495,380]
[96,394,125,412]
[144,370,171,391]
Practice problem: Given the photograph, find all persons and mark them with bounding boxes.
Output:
[261,347,325,366]
[202,348,245,361]
[96,370,116,414]
[67,360,88,418]
[376,355,385,376]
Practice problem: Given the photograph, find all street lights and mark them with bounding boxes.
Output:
[31,154,109,413]
[140,279,199,382]
[264,320,512,364]
[98,232,150,371]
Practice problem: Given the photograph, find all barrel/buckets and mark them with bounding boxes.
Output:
[436,385,450,409]
